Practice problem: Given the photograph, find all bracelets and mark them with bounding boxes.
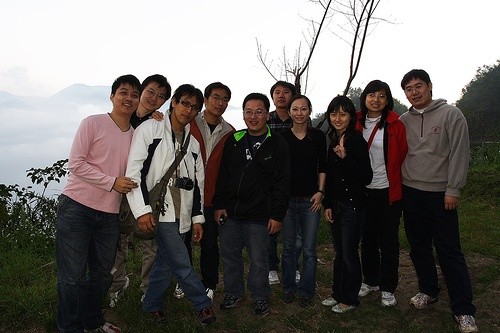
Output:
[318,190,324,194]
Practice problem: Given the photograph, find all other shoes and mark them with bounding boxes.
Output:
[139,284,148,303]
[298,295,313,307]
[331,302,356,313]
[322,296,338,306]
[267,271,280,285]
[294,271,300,280]
[204,286,216,303]
[172,281,184,299]
[282,289,295,304]
[109,276,129,308]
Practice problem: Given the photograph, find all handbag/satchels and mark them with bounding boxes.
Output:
[118,179,167,240]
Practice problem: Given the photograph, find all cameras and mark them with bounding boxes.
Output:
[220,215,226,225]
[174,177,193,190]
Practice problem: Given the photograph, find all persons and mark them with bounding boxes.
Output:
[279,95,327,307]
[151,81,235,301]
[322,95,374,313]
[211,93,290,313]
[265,81,312,285]
[124,84,218,324]
[354,80,408,308]
[54,75,139,333]
[108,74,171,308]
[398,70,478,333]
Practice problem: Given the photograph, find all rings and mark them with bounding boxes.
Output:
[337,145,338,148]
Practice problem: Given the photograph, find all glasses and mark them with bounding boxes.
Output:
[145,88,165,102]
[179,98,200,112]
[366,93,386,100]
[243,109,265,118]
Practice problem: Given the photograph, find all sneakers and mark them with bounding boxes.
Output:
[253,297,271,315]
[220,293,244,311]
[409,292,438,310]
[83,321,122,333]
[381,290,397,307]
[197,306,216,324]
[357,282,380,297]
[454,313,478,333]
[148,310,168,325]
